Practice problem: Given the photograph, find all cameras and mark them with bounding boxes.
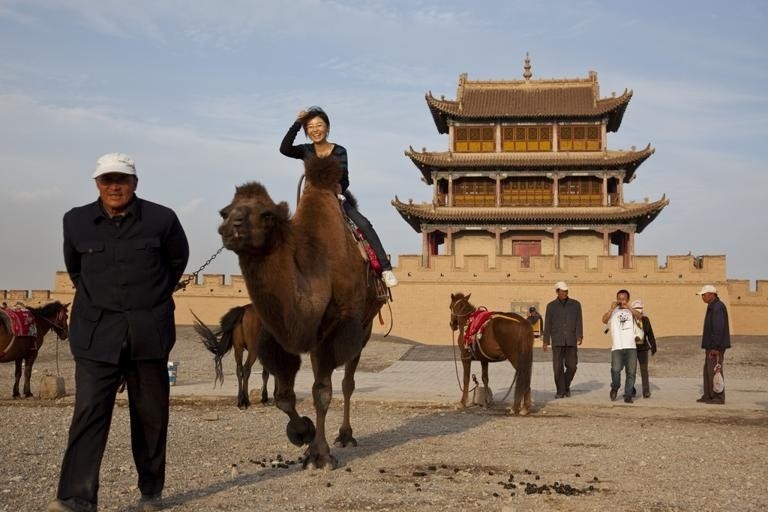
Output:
[617,301,622,307]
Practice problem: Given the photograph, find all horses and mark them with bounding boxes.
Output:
[1,298,71,400]
[190,302,279,411]
[449,292,535,417]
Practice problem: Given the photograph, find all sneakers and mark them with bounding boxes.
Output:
[381,270,398,289]
[555,388,571,398]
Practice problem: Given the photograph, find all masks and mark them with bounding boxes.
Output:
[634,309,643,314]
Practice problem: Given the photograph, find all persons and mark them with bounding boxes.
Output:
[527,306,543,338]
[280,105,400,287]
[631,300,657,398]
[543,282,583,399]
[602,289,642,402]
[696,285,730,405]
[57,152,190,510]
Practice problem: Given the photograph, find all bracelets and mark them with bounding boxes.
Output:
[608,309,613,313]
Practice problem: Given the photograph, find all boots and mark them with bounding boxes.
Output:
[48,497,96,512]
[137,491,163,510]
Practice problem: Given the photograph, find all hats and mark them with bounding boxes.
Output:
[696,285,717,295]
[554,281,569,292]
[631,300,644,308]
[92,153,136,177]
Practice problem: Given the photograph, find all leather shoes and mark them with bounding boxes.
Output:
[609,387,651,403]
[696,395,724,404]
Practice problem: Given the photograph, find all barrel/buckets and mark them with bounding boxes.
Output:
[168,362,179,385]
